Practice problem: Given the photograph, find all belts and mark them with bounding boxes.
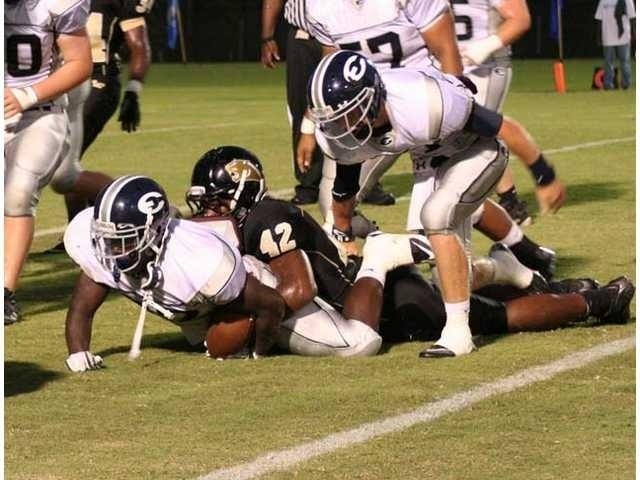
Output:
[26,105,60,113]
[413,135,477,170]
[484,53,511,64]
[93,64,118,73]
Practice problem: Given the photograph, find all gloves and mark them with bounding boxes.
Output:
[68,349,102,371]
[118,77,145,133]
[463,35,502,67]
[202,337,257,361]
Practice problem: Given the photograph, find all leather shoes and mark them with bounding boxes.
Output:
[293,193,315,203]
[366,185,395,203]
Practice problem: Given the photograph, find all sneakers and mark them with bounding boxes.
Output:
[369,221,378,233]
[54,240,64,249]
[494,241,549,294]
[527,247,554,278]
[4,287,20,325]
[420,329,475,358]
[603,274,636,325]
[364,230,434,265]
[570,276,598,294]
[500,198,531,226]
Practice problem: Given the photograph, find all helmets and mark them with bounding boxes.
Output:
[89,174,169,293]
[306,50,385,151]
[186,144,265,230]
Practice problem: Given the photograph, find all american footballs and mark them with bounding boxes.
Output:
[204,313,255,360]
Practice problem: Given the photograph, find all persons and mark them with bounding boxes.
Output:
[185,146,635,359]
[296,50,564,360]
[594,0,636,90]
[257,1,398,206]
[3,0,98,307]
[67,175,549,371]
[297,0,463,236]
[45,0,149,254]
[436,0,530,232]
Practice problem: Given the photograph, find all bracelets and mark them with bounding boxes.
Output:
[260,36,275,45]
